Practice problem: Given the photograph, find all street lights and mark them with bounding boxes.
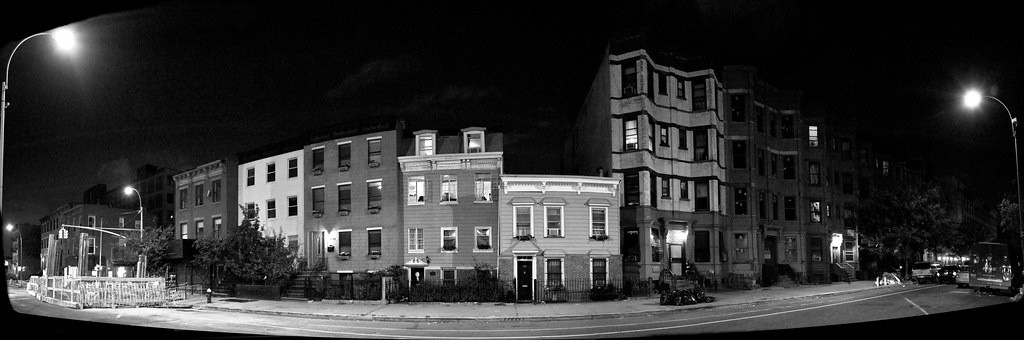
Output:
[0,27,80,218]
[124,186,142,240]
[7,224,23,287]
[965,90,1022,245]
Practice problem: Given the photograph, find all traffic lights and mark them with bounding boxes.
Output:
[58,229,69,240]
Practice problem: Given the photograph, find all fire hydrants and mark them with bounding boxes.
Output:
[205,288,212,303]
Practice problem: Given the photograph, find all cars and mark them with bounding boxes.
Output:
[937,261,969,288]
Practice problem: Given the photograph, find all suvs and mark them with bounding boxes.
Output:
[911,262,942,283]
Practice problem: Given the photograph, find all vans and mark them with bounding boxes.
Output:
[970,241,1021,295]
